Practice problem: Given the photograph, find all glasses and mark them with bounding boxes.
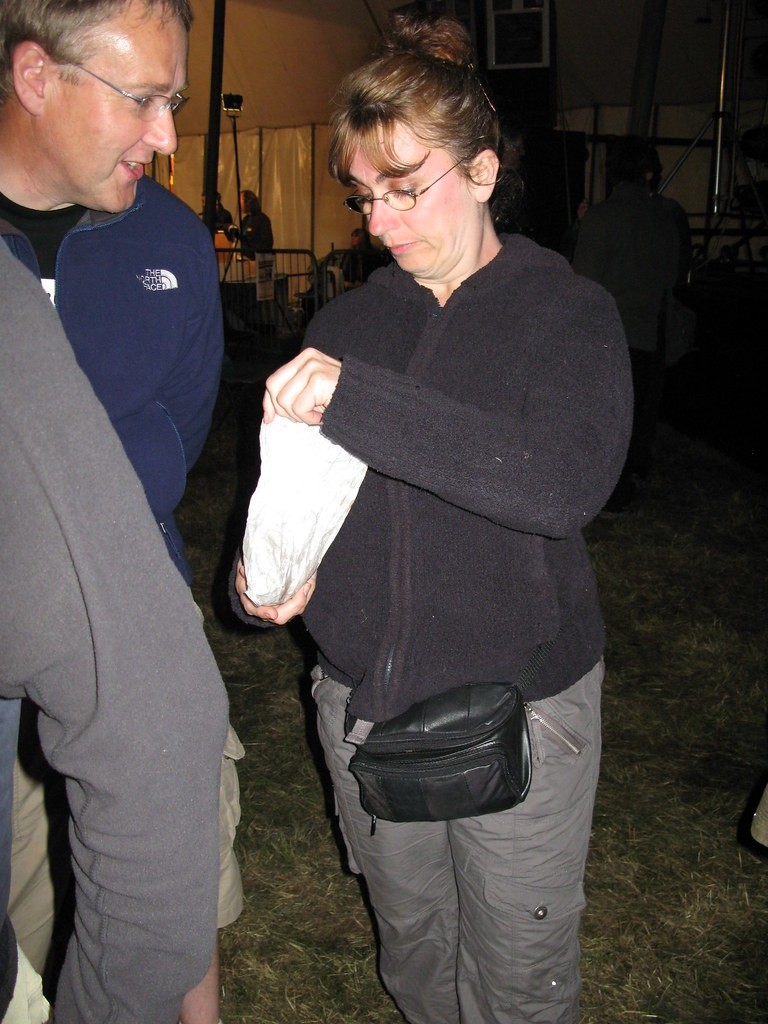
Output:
[340,152,469,217]
[52,50,191,123]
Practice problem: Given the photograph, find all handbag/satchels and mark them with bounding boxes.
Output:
[340,678,534,838]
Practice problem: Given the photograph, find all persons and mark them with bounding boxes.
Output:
[226,10,634,1024]
[569,134,690,520]
[0,0,274,1024]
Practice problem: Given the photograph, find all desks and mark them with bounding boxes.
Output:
[658,273,768,480]
[220,274,288,328]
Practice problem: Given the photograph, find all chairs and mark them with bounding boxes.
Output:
[213,329,270,431]
